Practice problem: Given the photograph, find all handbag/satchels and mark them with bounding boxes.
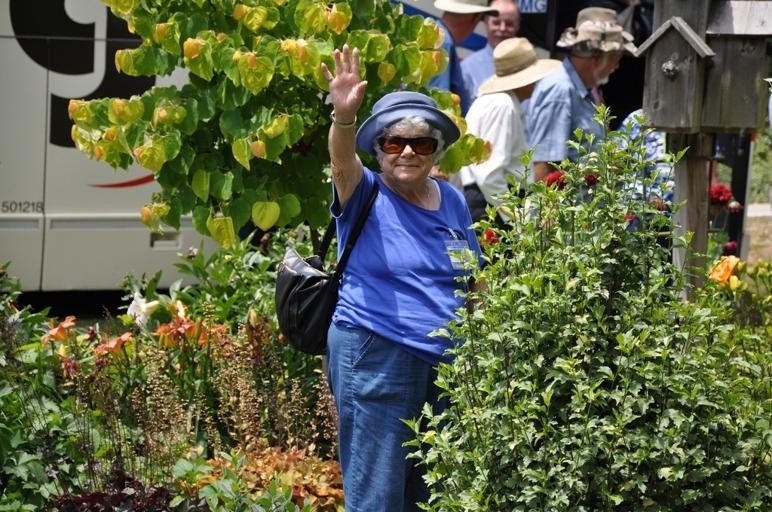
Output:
[274,247,338,355]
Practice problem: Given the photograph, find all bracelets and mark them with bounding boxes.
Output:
[329,109,358,129]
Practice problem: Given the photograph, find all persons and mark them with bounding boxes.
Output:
[320,43,491,512]
[400,1,626,272]
[611,108,726,271]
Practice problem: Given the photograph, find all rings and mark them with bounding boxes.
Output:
[328,77,333,81]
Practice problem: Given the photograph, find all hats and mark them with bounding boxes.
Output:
[557,8,640,57]
[432,0,500,17]
[355,90,461,152]
[477,37,562,94]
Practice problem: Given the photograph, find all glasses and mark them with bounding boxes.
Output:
[376,134,439,155]
